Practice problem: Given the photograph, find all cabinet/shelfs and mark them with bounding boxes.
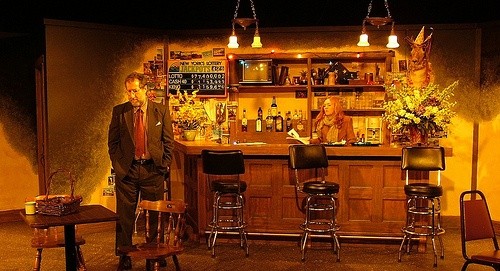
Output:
[225,50,395,143]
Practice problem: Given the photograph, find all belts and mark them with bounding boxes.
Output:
[133,160,153,165]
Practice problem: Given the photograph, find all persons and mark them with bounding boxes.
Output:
[310,96,356,143]
[108,71,175,271]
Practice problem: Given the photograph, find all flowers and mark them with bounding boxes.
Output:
[168,88,208,130]
[378,74,459,138]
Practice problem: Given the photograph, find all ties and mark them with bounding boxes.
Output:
[134,108,144,158]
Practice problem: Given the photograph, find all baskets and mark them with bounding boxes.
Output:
[36,169,83,215]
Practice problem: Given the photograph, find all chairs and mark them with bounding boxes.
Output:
[460,190,500,271]
[25,194,87,271]
[116,199,189,271]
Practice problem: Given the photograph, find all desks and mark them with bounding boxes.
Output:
[19,205,119,271]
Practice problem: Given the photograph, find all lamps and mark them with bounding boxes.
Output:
[227,0,263,48]
[356,0,400,48]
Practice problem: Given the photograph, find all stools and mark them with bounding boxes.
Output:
[201,150,250,258]
[398,146,446,267]
[289,144,341,262]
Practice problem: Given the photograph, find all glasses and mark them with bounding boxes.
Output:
[126,86,143,95]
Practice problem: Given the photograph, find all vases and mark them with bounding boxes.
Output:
[182,130,197,141]
[407,126,428,146]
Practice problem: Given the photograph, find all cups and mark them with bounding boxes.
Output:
[24,202,39,214]
[364,73,373,85]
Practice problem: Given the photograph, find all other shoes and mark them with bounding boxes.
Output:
[118,255,131,270]
[145,257,166,267]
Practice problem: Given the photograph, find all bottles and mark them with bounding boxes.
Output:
[241,109,248,133]
[286,76,290,86]
[292,110,298,131]
[286,111,292,132]
[266,110,273,133]
[297,109,305,133]
[328,69,335,85]
[256,117,262,132]
[258,106,263,120]
[275,111,283,132]
[271,96,277,120]
